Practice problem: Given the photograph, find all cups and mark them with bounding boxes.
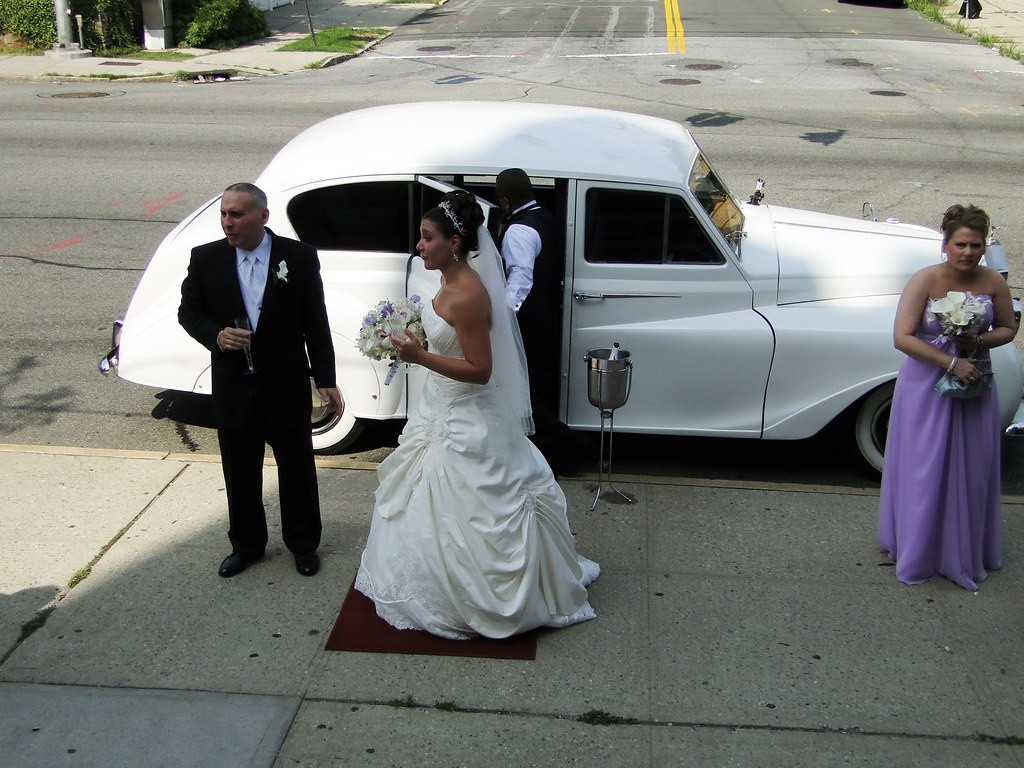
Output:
[233,317,258,375]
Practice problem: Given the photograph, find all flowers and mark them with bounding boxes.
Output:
[929,291,986,345]
[355,294,428,386]
[272,260,289,283]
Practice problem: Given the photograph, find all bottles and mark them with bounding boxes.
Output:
[606,342,620,360]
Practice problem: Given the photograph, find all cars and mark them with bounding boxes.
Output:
[100,102,1024,493]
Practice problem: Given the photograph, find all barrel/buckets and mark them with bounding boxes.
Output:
[584,350,631,409]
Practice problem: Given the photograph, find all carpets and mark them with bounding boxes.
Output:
[324,574,537,661]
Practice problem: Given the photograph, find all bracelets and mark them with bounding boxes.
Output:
[947,357,958,374]
[977,336,984,346]
[217,331,225,352]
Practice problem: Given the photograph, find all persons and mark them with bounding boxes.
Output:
[492,168,559,326]
[354,192,600,638]
[178,182,344,577]
[877,206,1017,593]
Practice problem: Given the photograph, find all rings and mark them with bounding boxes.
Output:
[969,377,975,383]
